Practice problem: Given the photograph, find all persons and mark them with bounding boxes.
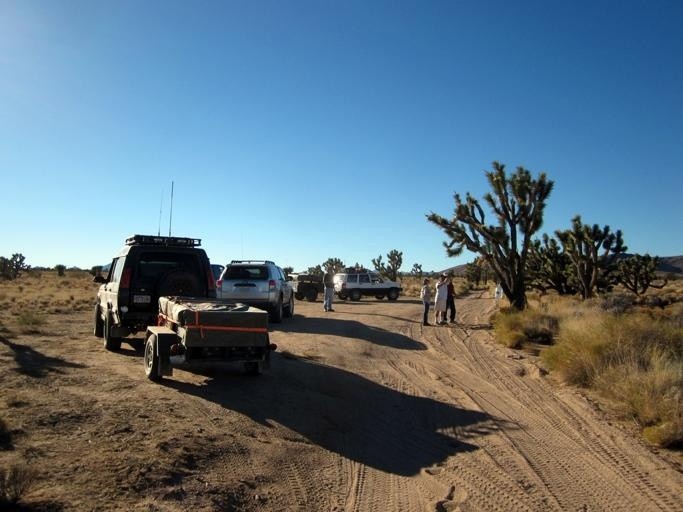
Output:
[444,274,456,323]
[434,275,450,326]
[323,266,335,312]
[420,279,433,326]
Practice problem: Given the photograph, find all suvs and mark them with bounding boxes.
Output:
[212,258,297,323]
[211,264,225,281]
[92,236,217,348]
[329,273,406,300]
